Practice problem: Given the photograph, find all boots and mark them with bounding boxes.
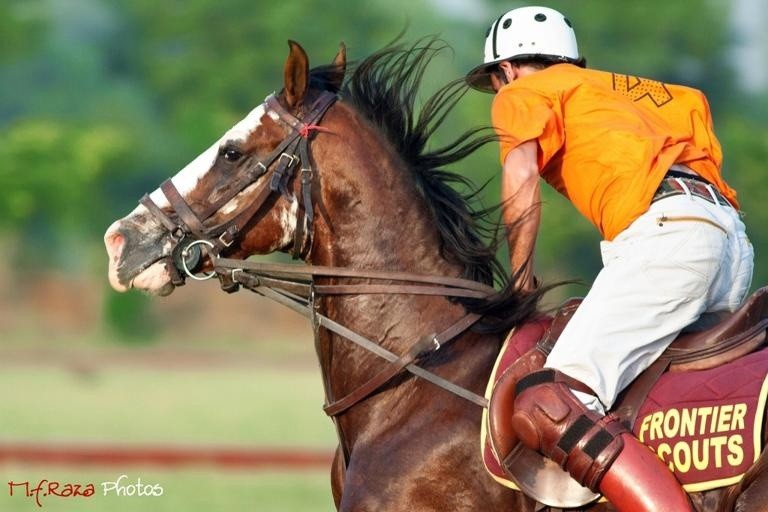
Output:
[554,406,699,511]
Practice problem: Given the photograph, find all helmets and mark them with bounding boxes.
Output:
[464,4,580,93]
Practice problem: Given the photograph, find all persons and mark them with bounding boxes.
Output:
[466,6,755,512]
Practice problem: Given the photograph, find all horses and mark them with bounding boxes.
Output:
[102,14,768,512]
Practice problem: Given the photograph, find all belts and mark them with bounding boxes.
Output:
[639,175,733,208]
